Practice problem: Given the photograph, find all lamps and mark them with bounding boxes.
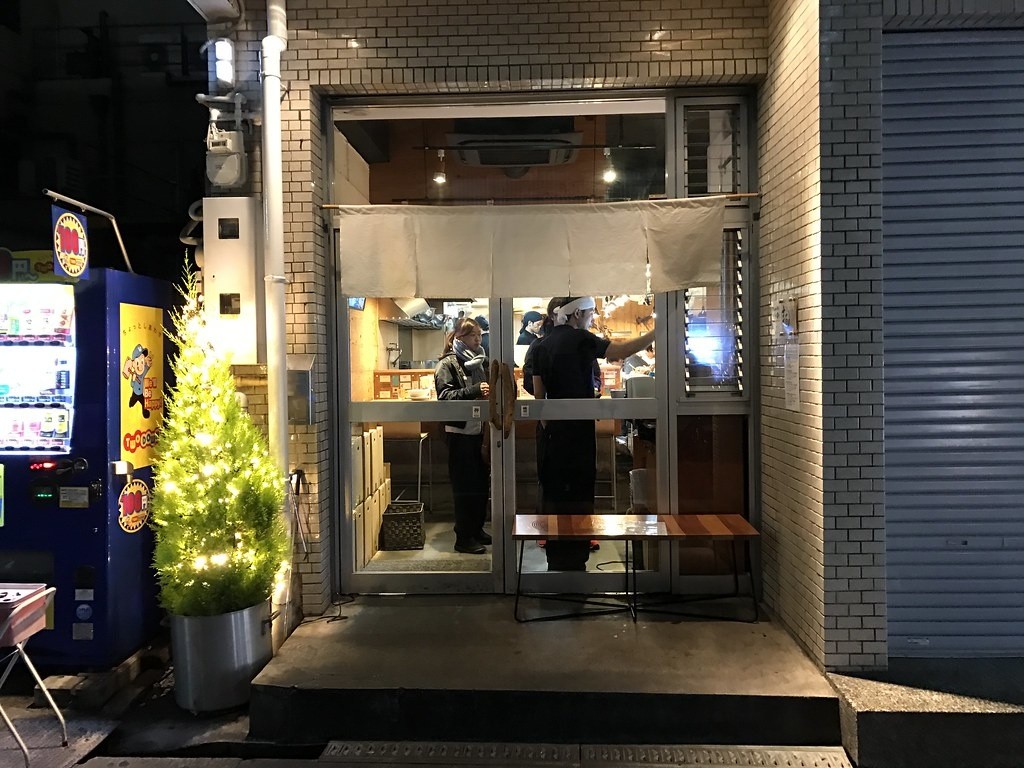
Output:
[602,148,616,182]
[433,149,447,183]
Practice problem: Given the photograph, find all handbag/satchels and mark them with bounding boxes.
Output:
[445,420,483,435]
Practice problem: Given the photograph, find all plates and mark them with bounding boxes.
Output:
[407,396,430,400]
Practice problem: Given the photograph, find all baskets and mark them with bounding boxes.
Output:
[382,503,426,550]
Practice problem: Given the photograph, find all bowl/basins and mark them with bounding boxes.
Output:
[610,389,625,398]
[407,389,429,397]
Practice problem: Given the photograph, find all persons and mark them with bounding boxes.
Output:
[434,318,490,554]
[475,296,656,572]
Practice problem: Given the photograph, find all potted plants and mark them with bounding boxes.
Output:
[146,249,291,710]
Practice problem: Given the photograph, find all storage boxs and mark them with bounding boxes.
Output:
[0,583,47,646]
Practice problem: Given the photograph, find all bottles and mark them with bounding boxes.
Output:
[54,360,72,395]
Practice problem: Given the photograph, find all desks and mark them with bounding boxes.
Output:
[512,515,761,623]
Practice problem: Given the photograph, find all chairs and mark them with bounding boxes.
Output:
[377,421,432,512]
[0,587,68,768]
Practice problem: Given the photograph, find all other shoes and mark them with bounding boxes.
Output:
[477,529,492,545]
[590,540,599,550]
[454,539,486,554]
[536,539,547,547]
